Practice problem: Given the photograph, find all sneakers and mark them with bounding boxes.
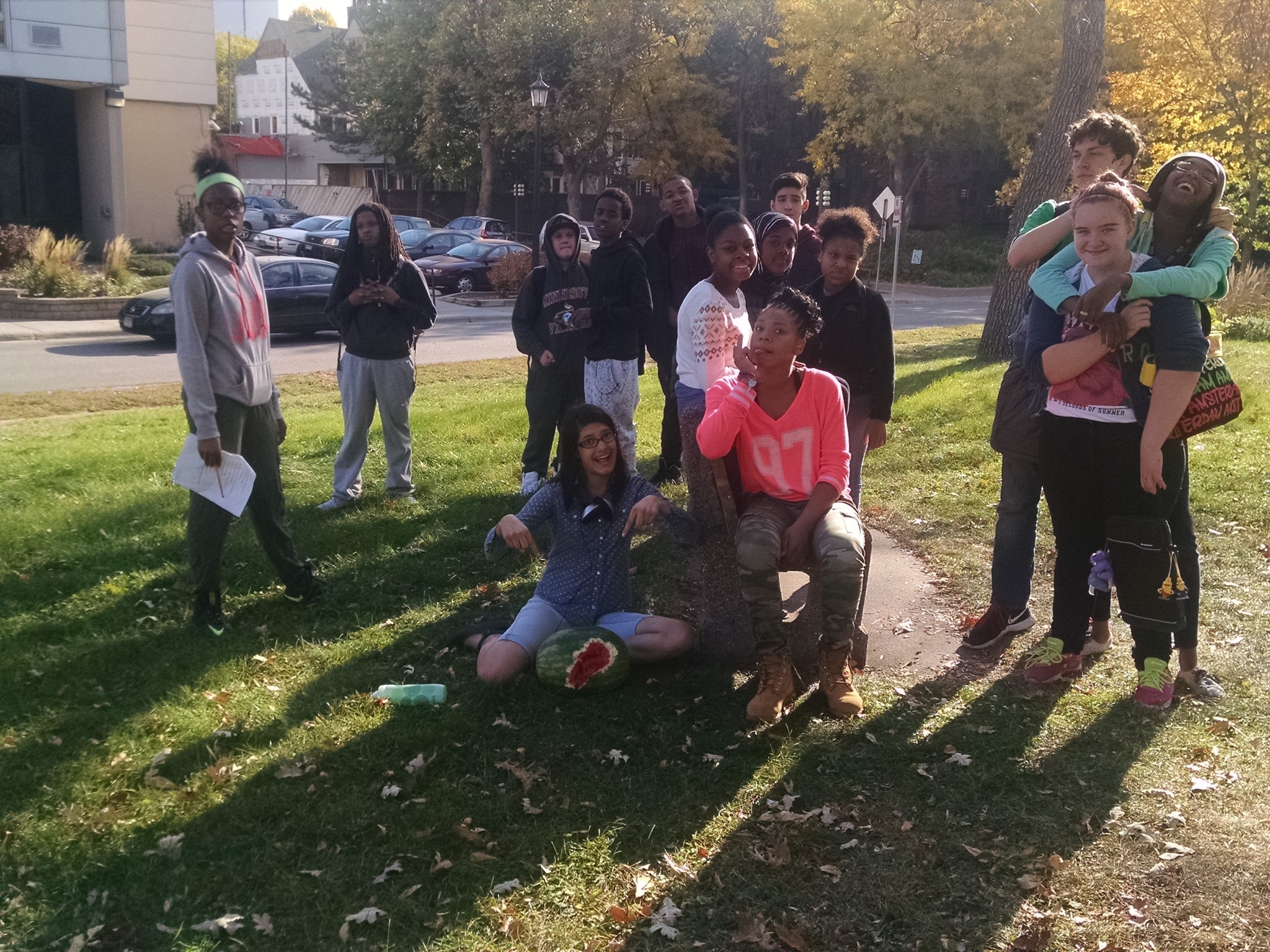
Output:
[1025,637,1083,683]
[1135,656,1174,710]
[283,576,328,602]
[194,591,226,640]
[1026,628,1052,657]
[962,601,1035,650]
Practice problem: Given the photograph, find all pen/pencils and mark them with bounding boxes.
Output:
[213,463,225,498]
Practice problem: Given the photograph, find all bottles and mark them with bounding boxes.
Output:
[372,683,447,707]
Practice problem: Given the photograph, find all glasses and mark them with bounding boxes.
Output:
[579,431,618,448]
[1173,159,1218,184]
[203,200,247,215]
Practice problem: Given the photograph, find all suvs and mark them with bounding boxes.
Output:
[304,213,432,265]
[538,219,624,265]
[442,215,515,242]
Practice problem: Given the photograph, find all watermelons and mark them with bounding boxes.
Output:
[536,626,629,695]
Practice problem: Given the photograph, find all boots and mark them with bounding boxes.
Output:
[817,634,864,717]
[747,644,796,724]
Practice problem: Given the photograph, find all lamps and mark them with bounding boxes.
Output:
[104,88,126,109]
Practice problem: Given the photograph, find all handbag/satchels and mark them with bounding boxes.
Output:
[1114,257,1244,439]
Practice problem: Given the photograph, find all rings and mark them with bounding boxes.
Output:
[1080,310,1088,316]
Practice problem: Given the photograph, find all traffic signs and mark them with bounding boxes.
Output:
[892,195,902,228]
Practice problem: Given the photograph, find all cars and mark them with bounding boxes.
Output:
[400,226,489,261]
[411,238,532,293]
[253,214,352,255]
[117,256,341,346]
[237,195,313,241]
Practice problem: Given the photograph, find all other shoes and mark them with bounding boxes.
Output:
[649,457,684,487]
[444,617,510,654]
[551,463,561,477]
[317,498,353,511]
[389,494,419,507]
[1081,634,1113,657]
[520,471,544,497]
[1174,667,1226,700]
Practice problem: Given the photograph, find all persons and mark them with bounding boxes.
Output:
[767,173,822,291]
[675,202,759,528]
[741,210,799,332]
[961,111,1239,712]
[465,404,703,686]
[316,202,438,512]
[512,214,592,498]
[568,186,653,480]
[696,288,867,722]
[643,174,713,488]
[168,148,331,640]
[800,207,896,511]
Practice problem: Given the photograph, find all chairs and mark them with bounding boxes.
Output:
[680,399,875,670]
[265,271,325,286]
[497,248,506,258]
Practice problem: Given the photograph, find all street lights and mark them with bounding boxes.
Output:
[527,70,551,268]
[512,175,525,241]
[284,23,322,199]
